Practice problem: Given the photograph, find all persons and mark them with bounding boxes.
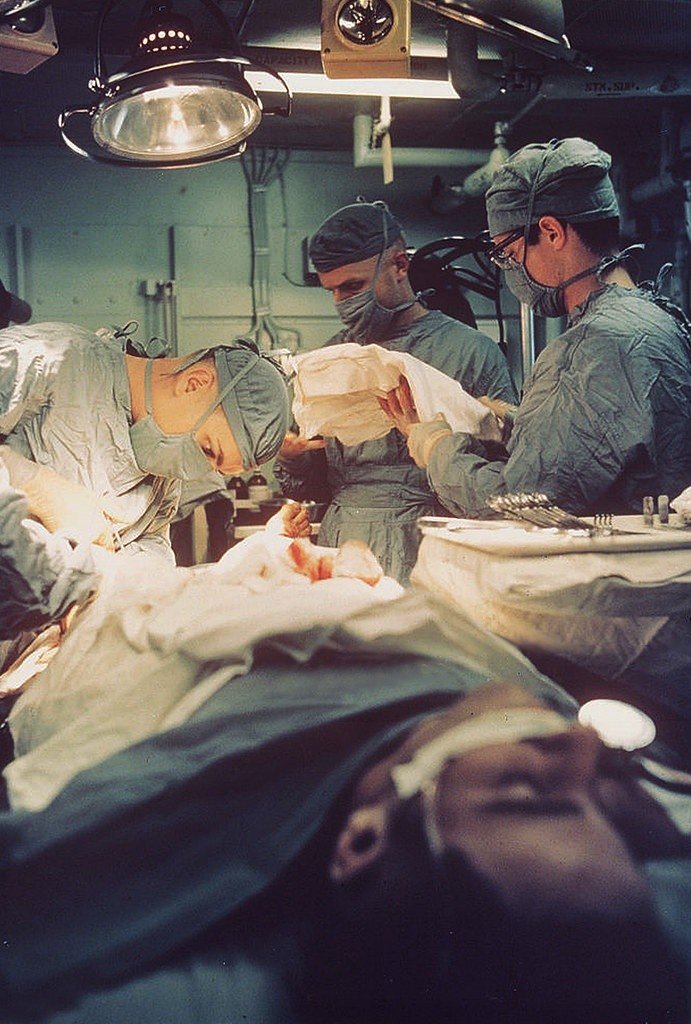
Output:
[406,138,691,519]
[272,203,517,588]
[78,501,690,1024]
[0,277,32,329]
[0,321,289,566]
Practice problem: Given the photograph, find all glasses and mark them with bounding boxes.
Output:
[486,229,526,269]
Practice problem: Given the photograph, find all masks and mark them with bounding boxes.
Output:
[503,250,561,318]
[130,395,223,483]
[335,279,402,344]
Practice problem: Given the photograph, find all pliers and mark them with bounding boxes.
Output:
[484,490,652,534]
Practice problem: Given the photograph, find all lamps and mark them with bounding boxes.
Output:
[42,0,293,171]
[0,0,59,75]
[319,0,412,76]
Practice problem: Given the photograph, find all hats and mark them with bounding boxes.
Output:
[211,338,289,474]
[307,203,401,274]
[485,138,619,235]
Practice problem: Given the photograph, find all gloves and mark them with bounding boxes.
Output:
[476,396,513,421]
[14,465,131,552]
[404,414,453,470]
